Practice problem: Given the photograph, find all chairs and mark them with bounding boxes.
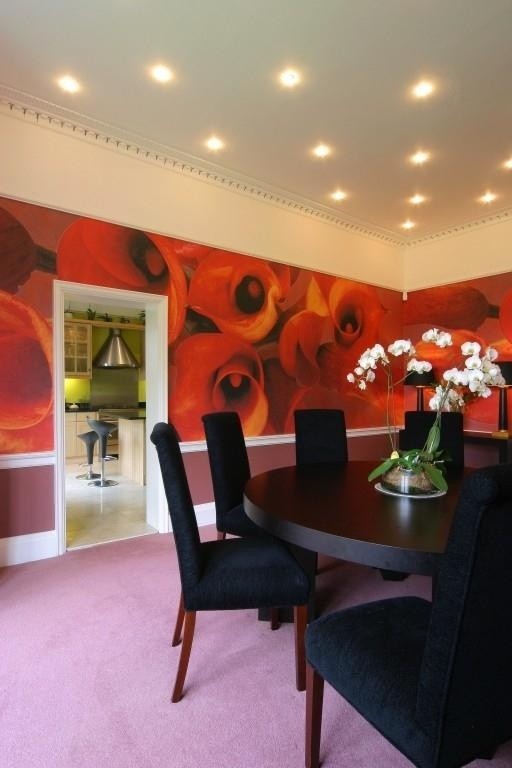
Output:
[305,466,512,766]
[149,423,311,704]
[294,406,345,465]
[401,410,466,464]
[201,409,256,541]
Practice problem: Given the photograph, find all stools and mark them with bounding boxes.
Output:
[75,420,119,487]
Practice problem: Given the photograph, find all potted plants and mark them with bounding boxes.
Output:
[86,308,130,324]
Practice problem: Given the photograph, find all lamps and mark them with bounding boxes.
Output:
[487,361,512,438]
[402,370,435,412]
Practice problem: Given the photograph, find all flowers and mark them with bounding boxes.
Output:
[346,326,507,493]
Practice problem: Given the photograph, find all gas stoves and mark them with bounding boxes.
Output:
[92,403,139,410]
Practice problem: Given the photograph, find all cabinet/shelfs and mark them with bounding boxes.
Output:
[66,413,98,457]
[64,321,93,379]
